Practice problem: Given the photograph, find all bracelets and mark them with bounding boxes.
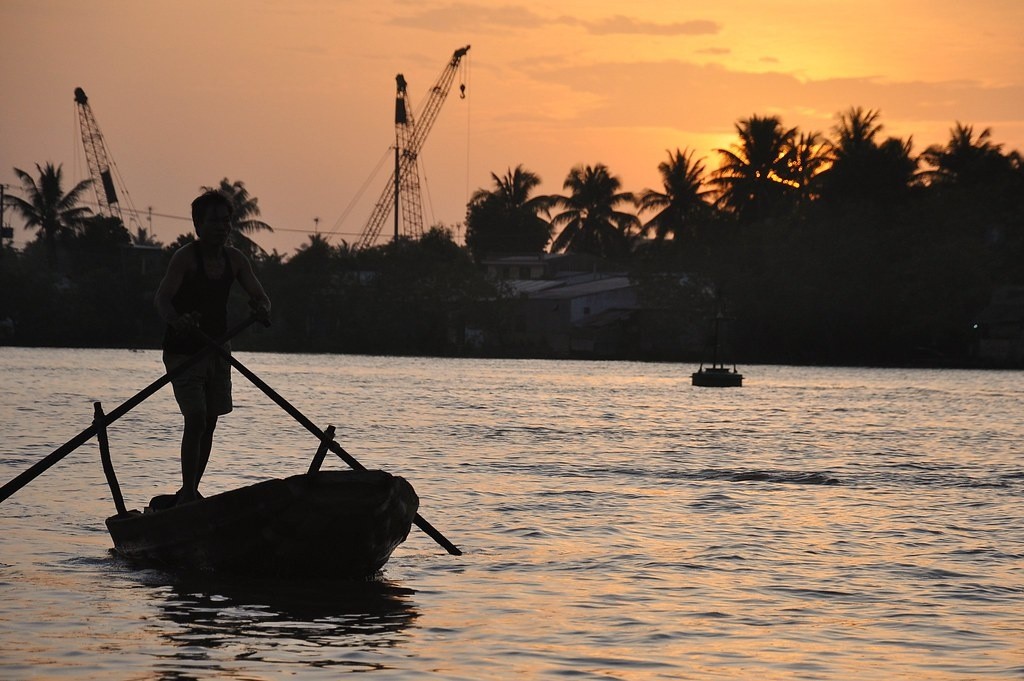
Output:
[256,303,268,317]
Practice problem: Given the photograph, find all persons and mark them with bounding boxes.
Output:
[153,190,273,503]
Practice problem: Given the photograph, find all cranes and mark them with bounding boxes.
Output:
[325,40,476,251]
[392,68,435,248]
[71,86,145,239]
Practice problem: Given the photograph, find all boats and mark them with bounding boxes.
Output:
[689,305,748,391]
[103,464,422,602]
